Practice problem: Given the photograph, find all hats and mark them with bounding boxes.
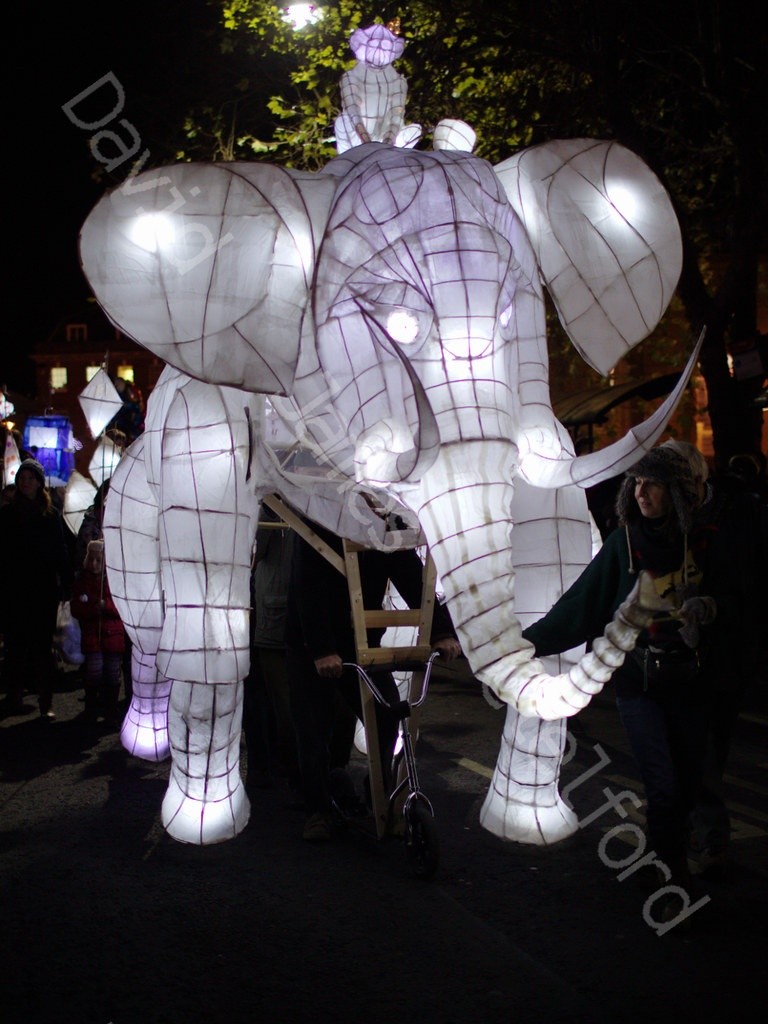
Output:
[82,538,107,570]
[15,458,45,490]
[616,439,707,533]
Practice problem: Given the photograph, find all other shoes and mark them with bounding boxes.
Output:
[79,711,100,742]
[0,695,22,709]
[330,767,361,801]
[102,716,120,734]
[282,779,306,811]
[663,885,710,924]
[303,811,330,839]
[39,704,56,721]
[388,795,410,841]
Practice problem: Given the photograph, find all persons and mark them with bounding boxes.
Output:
[574,434,614,492]
[243,498,300,790]
[0,459,76,721]
[295,538,461,839]
[70,538,125,714]
[77,478,110,544]
[660,440,768,873]
[522,448,723,925]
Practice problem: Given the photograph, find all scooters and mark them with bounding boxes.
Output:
[320,651,446,883]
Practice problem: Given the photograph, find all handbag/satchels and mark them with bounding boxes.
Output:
[87,656,103,672]
